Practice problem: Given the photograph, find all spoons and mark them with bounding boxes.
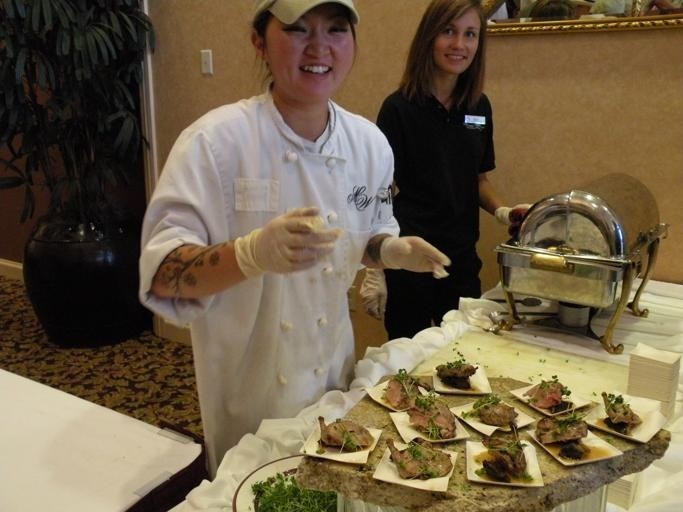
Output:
[488,298,542,307]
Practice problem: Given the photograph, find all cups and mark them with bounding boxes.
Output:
[557,301,590,328]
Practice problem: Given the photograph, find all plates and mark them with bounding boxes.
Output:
[432,365,493,395]
[366,379,440,412]
[583,393,666,444]
[527,429,623,467]
[374,442,459,493]
[625,363,667,416]
[608,470,639,510]
[465,440,545,488]
[300,420,382,465]
[449,398,535,437]
[236,453,349,512]
[389,412,471,445]
[510,386,589,417]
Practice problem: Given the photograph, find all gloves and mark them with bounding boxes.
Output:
[235,207,339,278]
[495,203,533,224]
[380,235,452,279]
[360,267,388,322]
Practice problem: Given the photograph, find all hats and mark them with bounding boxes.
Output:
[251,0,361,27]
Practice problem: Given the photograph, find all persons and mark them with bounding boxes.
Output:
[355,0,537,346]
[131,2,452,482]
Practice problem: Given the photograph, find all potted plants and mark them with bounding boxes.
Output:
[1,1,156,351]
